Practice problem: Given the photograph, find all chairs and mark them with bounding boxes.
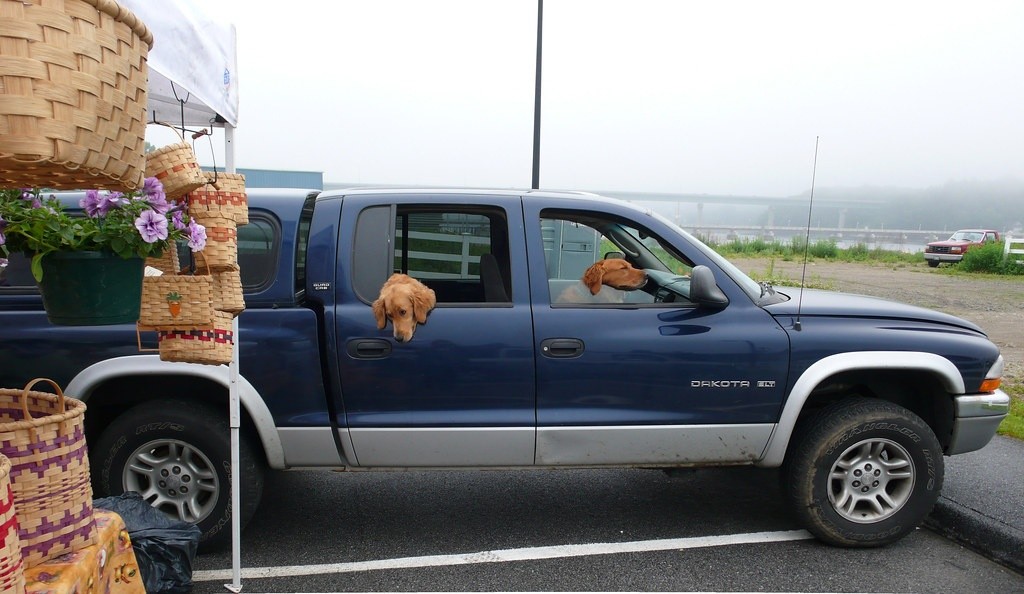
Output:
[479,253,510,302]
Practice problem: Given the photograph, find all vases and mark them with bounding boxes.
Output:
[25,250,148,326]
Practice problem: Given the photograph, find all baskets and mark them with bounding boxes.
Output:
[0,1,248,366]
[0,378,98,594]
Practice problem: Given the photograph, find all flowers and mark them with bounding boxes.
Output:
[0,177,209,284]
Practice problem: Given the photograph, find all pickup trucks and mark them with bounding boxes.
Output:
[924,229,1000,268]
[0,187,1012,553]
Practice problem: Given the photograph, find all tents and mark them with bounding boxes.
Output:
[118,0,245,594]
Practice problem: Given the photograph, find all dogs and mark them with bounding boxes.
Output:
[372,272,436,344]
[554,257,650,304]
